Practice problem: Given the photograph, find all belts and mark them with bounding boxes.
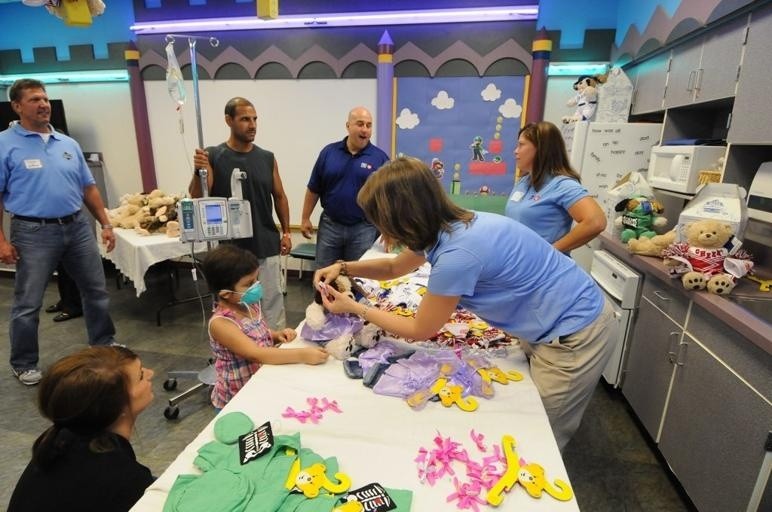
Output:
[11,208,84,226]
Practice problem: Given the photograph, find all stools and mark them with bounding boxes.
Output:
[284,240,317,294]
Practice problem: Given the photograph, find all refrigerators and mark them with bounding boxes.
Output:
[561,122,663,271]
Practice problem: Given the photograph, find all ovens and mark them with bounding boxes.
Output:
[591,249,645,388]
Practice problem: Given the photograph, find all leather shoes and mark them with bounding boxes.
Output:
[53,312,74,322]
[45,304,63,313]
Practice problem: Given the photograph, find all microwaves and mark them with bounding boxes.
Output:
[646,145,726,194]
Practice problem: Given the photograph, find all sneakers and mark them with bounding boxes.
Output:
[11,366,43,386]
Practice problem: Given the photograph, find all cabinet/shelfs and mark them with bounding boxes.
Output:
[725,0,772,146]
[662,11,750,111]
[621,276,772,512]
[622,48,672,116]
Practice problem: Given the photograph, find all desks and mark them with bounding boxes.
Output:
[96,204,218,327]
[125,232,580,512]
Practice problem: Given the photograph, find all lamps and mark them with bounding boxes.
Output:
[130,5,540,36]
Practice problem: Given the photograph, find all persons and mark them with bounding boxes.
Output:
[503,121,608,259]
[7,346,159,512]
[0,78,128,385]
[313,156,622,458]
[45,119,83,322]
[301,106,397,303]
[189,96,292,331]
[203,243,329,415]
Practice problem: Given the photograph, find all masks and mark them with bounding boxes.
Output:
[233,281,265,310]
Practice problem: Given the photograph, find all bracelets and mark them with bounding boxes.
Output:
[361,307,369,322]
[194,169,200,175]
[335,260,348,278]
[284,233,292,238]
[102,225,114,230]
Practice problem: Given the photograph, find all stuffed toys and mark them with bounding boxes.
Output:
[562,74,608,124]
[614,195,669,244]
[660,220,756,296]
[103,189,188,238]
[627,225,677,259]
